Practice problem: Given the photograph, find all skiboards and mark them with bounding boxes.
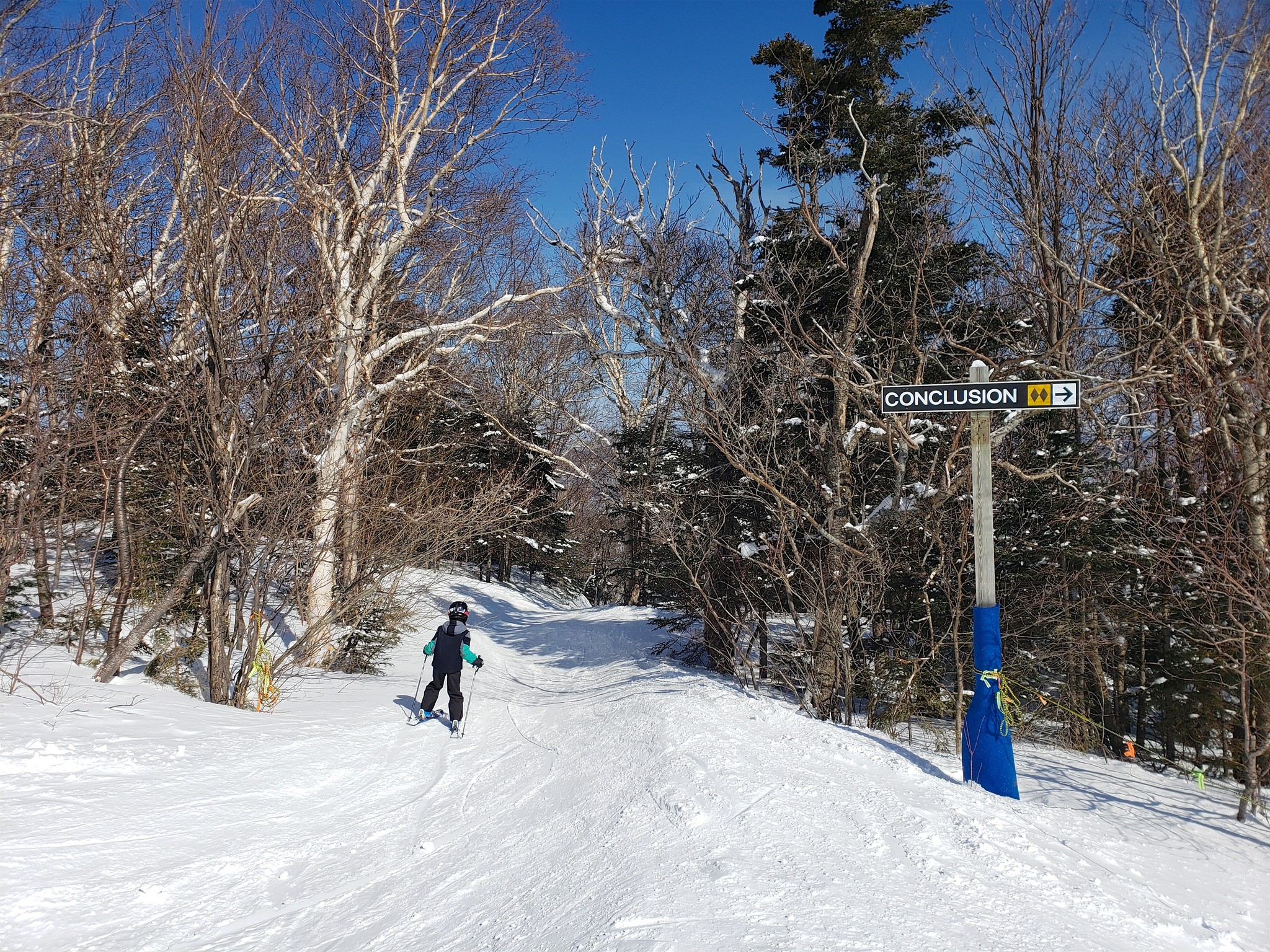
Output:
[405,709,459,739]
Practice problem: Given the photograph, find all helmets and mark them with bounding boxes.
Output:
[448,601,468,622]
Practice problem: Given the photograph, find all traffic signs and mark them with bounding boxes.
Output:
[880,380,1080,413]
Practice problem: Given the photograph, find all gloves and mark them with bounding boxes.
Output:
[470,658,484,668]
[423,644,431,656]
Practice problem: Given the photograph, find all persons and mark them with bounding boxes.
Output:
[418,601,484,731]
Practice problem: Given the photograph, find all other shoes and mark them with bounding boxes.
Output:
[451,720,461,728]
[422,710,432,719]
